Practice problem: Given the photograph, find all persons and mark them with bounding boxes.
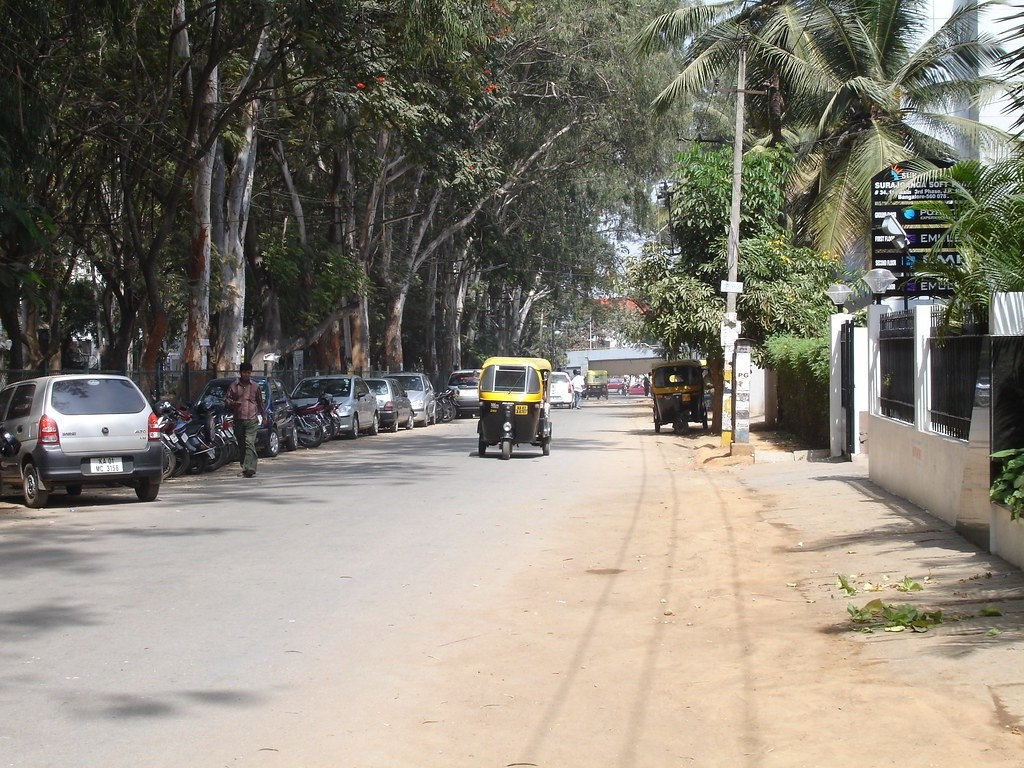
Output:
[225,362,268,477]
[570,369,577,380]
[622,378,628,391]
[572,370,585,409]
[644,377,649,397]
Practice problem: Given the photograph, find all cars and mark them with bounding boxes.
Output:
[548,371,576,409]
[188,376,300,456]
[382,372,436,427]
[288,374,381,437]
[0,374,164,509]
[364,379,417,433]
[608,376,626,390]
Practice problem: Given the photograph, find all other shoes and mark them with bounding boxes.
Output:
[576,406,580,409]
[243,469,254,478]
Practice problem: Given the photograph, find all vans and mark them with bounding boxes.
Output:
[445,369,483,417]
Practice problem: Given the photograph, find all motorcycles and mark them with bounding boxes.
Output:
[585,371,611,400]
[648,359,709,433]
[475,355,552,460]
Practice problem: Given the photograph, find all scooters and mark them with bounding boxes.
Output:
[148,388,240,480]
[432,387,463,424]
[294,392,342,450]
